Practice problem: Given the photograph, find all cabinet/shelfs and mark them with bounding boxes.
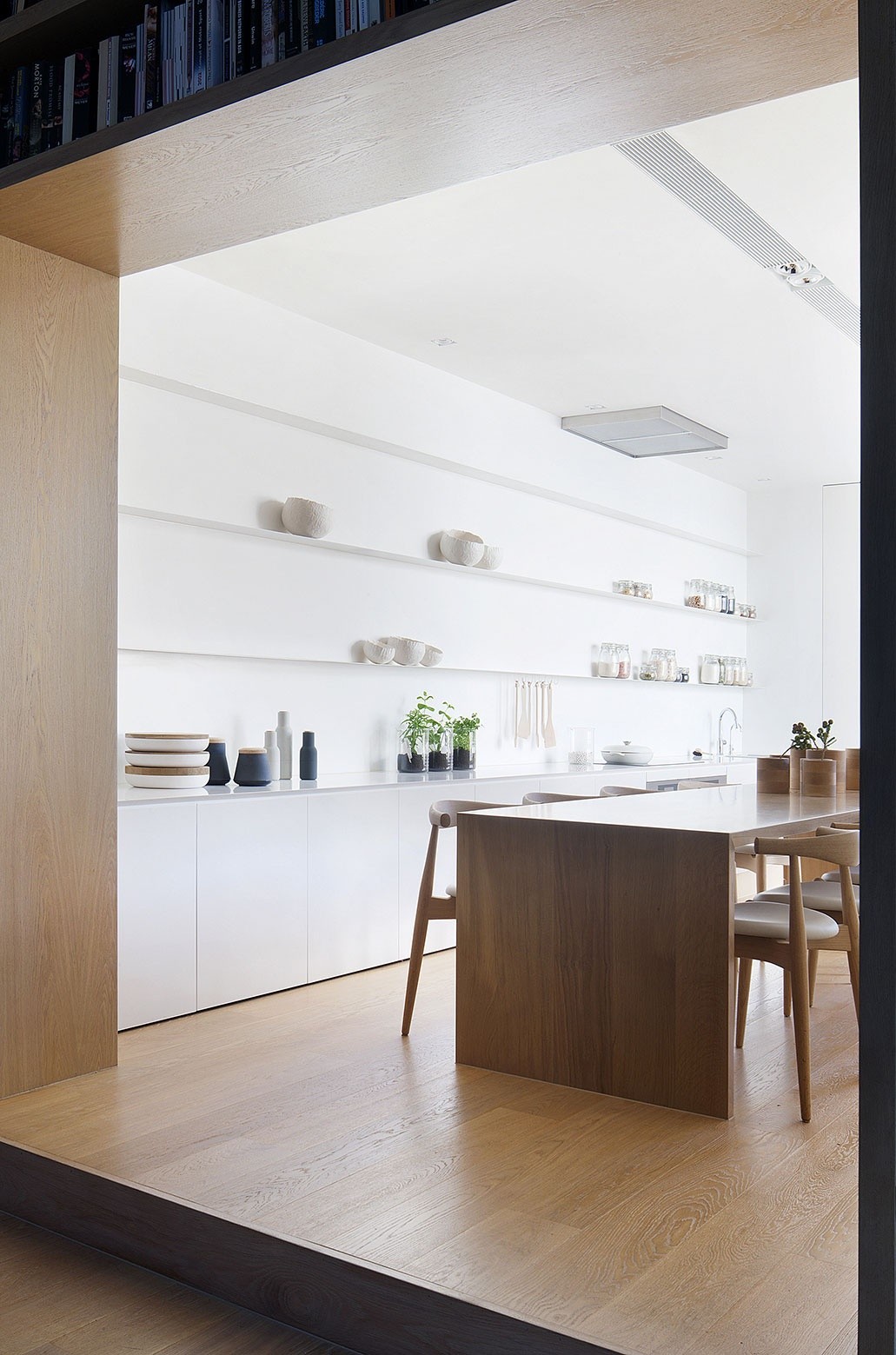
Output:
[1,1,859,278]
[119,364,760,813]
[117,763,756,1032]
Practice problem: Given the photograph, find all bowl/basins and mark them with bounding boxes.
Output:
[125,751,210,767]
[387,635,425,665]
[363,639,396,665]
[475,543,506,570]
[281,498,333,538]
[126,733,210,752]
[125,766,210,788]
[440,529,483,567]
[420,644,442,667]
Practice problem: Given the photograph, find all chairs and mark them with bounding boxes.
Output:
[733,822,860,1123]
[400,777,743,1039]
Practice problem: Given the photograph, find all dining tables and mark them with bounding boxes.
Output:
[455,777,860,1121]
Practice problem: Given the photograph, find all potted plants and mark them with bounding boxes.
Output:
[798,727,838,797]
[804,718,847,793]
[756,732,812,794]
[444,712,483,770]
[790,722,823,793]
[424,700,455,772]
[393,691,440,773]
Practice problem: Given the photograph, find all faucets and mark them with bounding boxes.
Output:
[727,721,742,755]
[717,707,739,756]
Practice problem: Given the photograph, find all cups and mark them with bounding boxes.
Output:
[233,748,272,787]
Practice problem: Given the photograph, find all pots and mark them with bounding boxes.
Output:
[601,740,653,765]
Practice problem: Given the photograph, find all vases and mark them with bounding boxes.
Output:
[844,748,859,792]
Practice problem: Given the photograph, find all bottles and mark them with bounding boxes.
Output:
[598,643,630,679]
[264,731,281,781]
[300,731,318,780]
[701,654,753,686]
[204,740,231,786]
[687,579,735,614]
[640,648,689,682]
[276,711,292,780]
